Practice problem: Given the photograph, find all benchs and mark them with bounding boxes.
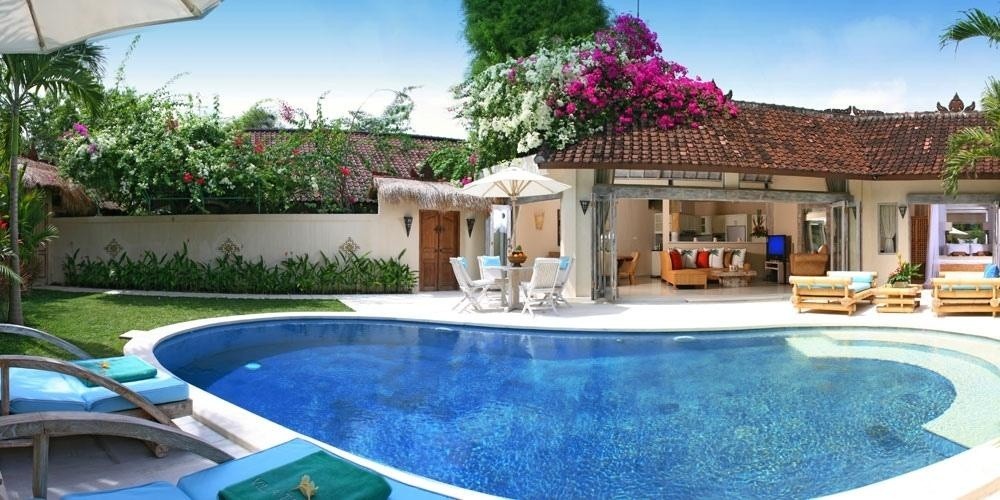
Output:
[931,271,1000,318]
[938,253,994,270]
[788,270,878,315]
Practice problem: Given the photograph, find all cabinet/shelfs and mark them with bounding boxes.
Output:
[654,212,725,234]
[765,262,785,284]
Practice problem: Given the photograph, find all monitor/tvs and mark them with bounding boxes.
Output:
[767,235,786,260]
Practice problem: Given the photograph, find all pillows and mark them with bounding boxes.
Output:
[984,264,999,278]
[669,248,748,269]
[971,244,991,253]
[946,244,969,256]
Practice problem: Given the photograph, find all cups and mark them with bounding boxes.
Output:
[728,265,737,272]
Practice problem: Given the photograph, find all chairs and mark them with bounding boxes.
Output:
[448,254,574,312]
[618,251,641,285]
[0,323,192,458]
[789,244,829,276]
[0,413,457,500]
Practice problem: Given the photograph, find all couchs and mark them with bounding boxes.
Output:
[660,252,753,289]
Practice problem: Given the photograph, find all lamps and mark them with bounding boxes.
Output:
[467,218,475,238]
[899,207,907,219]
[580,200,590,215]
[404,217,413,237]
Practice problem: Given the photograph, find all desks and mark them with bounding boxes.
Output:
[617,256,633,268]
[871,283,922,312]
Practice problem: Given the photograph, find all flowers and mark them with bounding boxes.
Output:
[886,255,923,285]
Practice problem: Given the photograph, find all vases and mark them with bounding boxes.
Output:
[895,282,909,288]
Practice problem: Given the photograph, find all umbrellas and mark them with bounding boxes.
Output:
[0,0,224,55]
[456,166,573,251]
